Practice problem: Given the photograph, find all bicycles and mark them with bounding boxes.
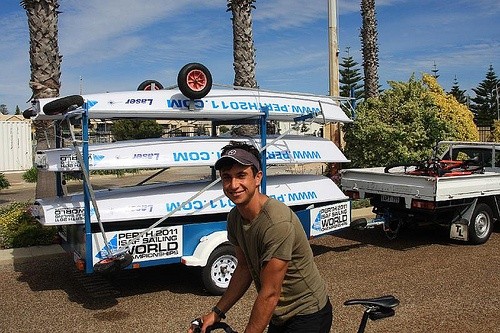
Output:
[188,295,400,333]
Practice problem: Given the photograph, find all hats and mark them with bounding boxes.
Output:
[214,148,262,172]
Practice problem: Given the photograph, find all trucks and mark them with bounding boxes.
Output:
[339,138,500,245]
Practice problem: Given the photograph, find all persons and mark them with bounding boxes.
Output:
[187,140,333,333]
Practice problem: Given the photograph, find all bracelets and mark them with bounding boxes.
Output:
[212,306,226,320]
